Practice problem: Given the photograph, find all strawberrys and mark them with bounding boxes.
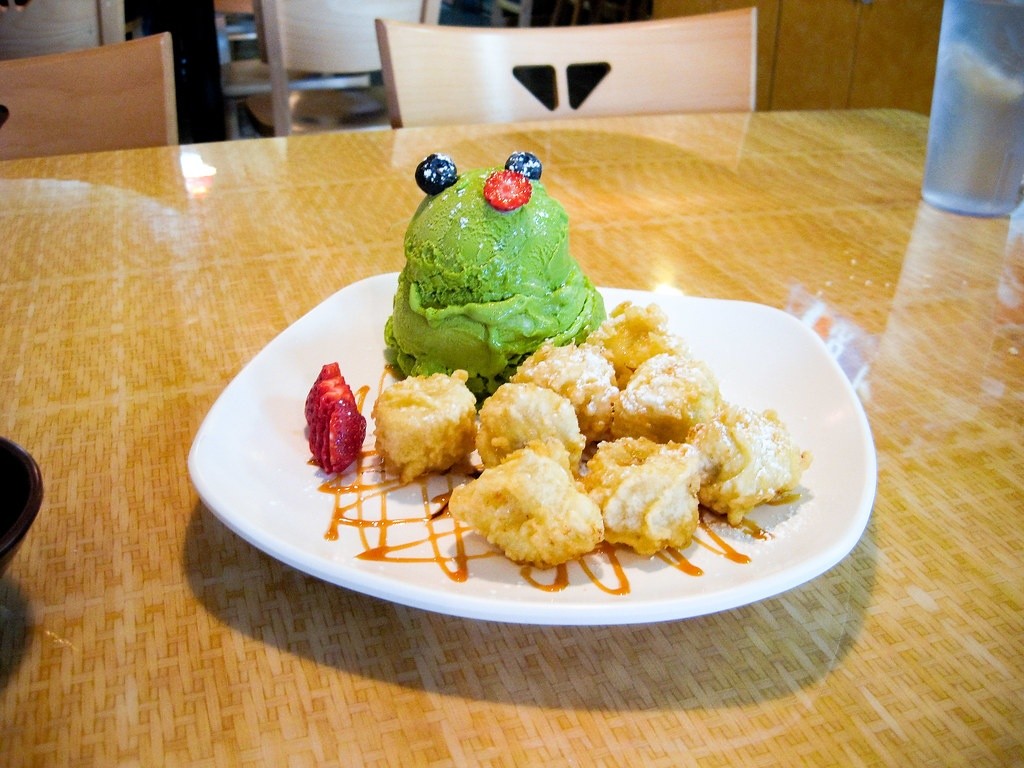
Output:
[305,362,367,474]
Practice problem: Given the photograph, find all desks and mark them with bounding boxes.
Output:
[0,107,1024,768]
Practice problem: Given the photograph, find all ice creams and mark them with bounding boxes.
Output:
[384,147,607,412]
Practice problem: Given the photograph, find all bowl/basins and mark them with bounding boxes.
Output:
[0,437,43,566]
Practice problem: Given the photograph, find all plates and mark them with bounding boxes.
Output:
[186,278,876,625]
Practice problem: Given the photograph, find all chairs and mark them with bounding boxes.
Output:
[0,0,757,161]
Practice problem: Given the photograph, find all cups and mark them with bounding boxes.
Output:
[919,0,1024,216]
